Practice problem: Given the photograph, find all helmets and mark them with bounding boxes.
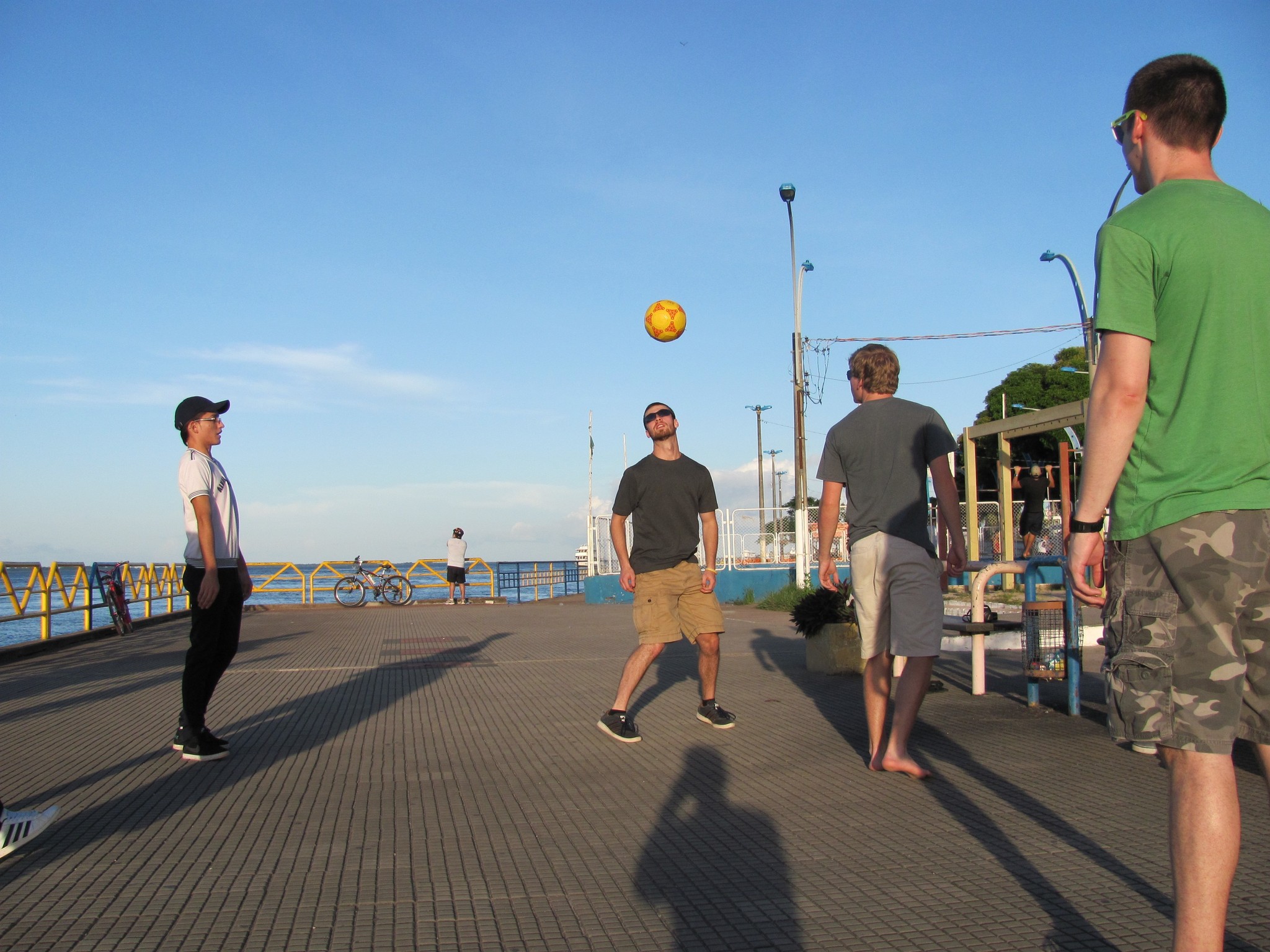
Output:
[453,528,464,536]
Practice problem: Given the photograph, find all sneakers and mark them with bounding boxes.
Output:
[182,732,229,761]
[461,600,466,604]
[444,599,455,605]
[1132,740,1158,754]
[597,710,642,742]
[0,804,62,858]
[172,727,229,752]
[696,700,735,728]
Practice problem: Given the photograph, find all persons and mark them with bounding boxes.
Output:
[174,396,255,761]
[595,402,736,743]
[444,527,471,605]
[1012,465,1056,559]
[813,343,970,776]
[1037,534,1052,556]
[991,530,1000,561]
[1066,50,1269,951]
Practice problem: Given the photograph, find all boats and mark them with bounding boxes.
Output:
[575,544,604,566]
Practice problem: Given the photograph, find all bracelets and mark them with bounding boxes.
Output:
[705,567,718,575]
[1066,510,1104,534]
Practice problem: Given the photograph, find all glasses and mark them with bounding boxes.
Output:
[1110,109,1147,145]
[644,409,673,427]
[189,417,221,429]
[847,369,858,380]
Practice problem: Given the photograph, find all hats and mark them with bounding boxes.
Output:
[175,396,230,430]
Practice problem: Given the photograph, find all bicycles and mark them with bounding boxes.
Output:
[99,560,134,635]
[334,554,412,608]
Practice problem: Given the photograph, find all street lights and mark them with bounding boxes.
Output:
[778,182,815,602]
[1012,250,1111,481]
[747,403,784,564]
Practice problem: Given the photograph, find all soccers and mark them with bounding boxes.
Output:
[644,300,687,343]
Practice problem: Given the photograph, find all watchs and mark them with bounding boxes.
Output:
[1014,473,1018,477]
